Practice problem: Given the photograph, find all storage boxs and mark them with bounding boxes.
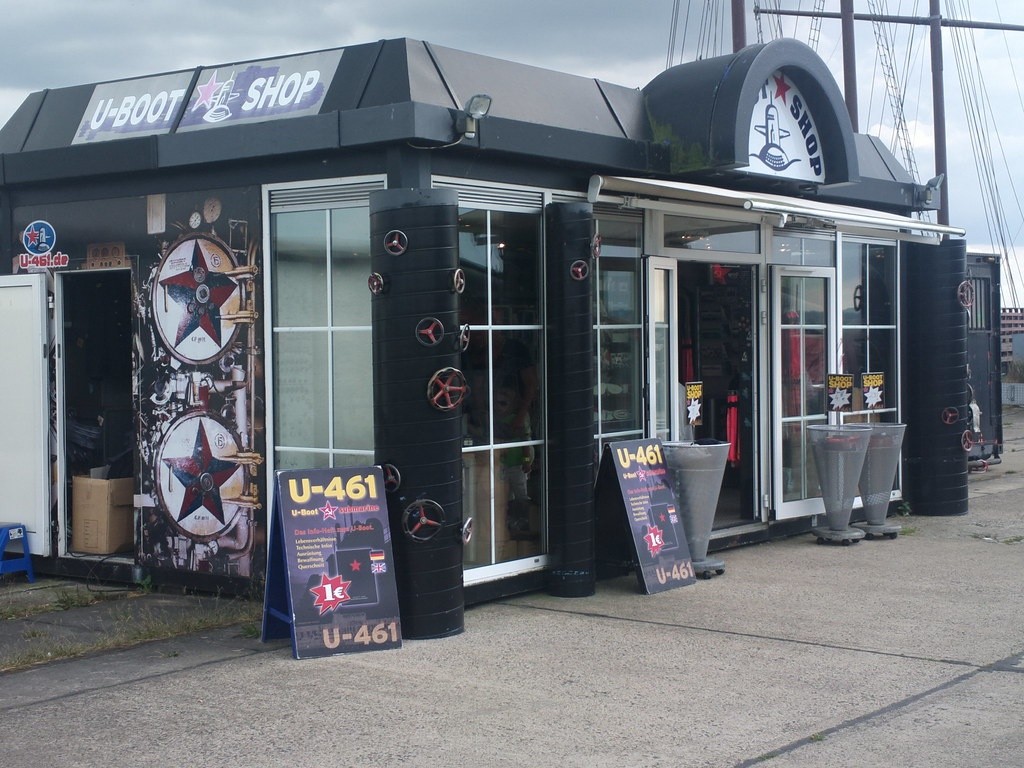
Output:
[71,473,133,555]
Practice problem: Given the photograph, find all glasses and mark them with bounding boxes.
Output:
[496,401,510,406]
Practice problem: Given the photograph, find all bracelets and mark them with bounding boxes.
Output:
[520,455,531,463]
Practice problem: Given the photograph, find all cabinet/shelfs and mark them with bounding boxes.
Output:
[695,286,725,376]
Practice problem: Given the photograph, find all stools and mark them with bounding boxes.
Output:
[0,522,35,585]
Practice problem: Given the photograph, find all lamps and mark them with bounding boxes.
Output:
[455,96,495,139]
[920,173,945,207]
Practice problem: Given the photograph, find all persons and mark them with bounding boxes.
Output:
[462,299,537,564]
